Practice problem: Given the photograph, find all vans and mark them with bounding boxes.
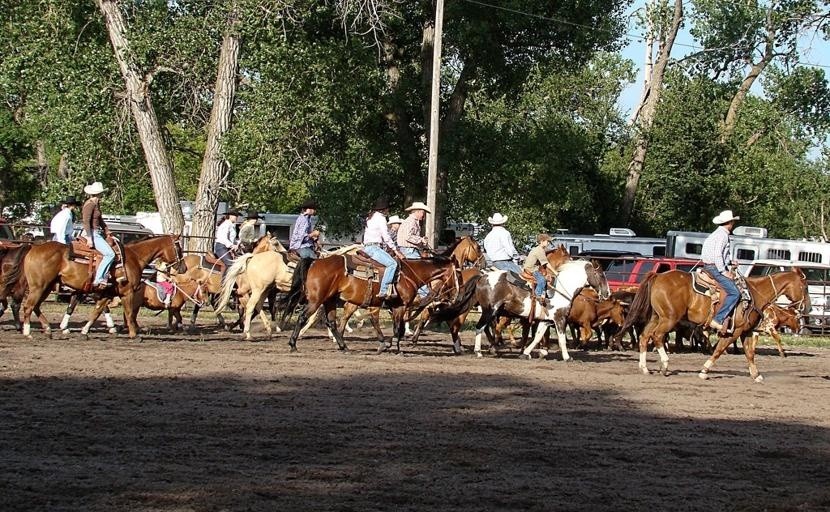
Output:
[570,248,704,294]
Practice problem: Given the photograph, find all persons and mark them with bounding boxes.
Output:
[361,198,404,298]
[288,201,320,259]
[397,201,432,260]
[523,234,559,303]
[381,215,404,258]
[239,209,264,253]
[48,200,76,245]
[50,196,82,296]
[214,207,244,309]
[700,209,741,333]
[0,215,19,244]
[484,212,524,274]
[82,180,120,286]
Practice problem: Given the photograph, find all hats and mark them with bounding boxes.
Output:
[222,208,243,216]
[245,210,264,218]
[298,200,321,210]
[387,215,404,225]
[405,202,431,214]
[712,210,741,224]
[537,234,556,242]
[84,181,109,194]
[372,198,397,210]
[487,213,509,225]
[59,196,83,206]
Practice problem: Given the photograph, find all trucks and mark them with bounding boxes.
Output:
[2,198,829,339]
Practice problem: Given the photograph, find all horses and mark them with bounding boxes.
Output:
[0,230,812,383]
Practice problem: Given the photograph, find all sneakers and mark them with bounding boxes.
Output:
[379,293,397,299]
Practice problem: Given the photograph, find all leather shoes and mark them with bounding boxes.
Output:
[93,281,113,288]
[709,320,731,333]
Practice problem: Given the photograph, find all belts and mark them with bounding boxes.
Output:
[365,242,383,249]
